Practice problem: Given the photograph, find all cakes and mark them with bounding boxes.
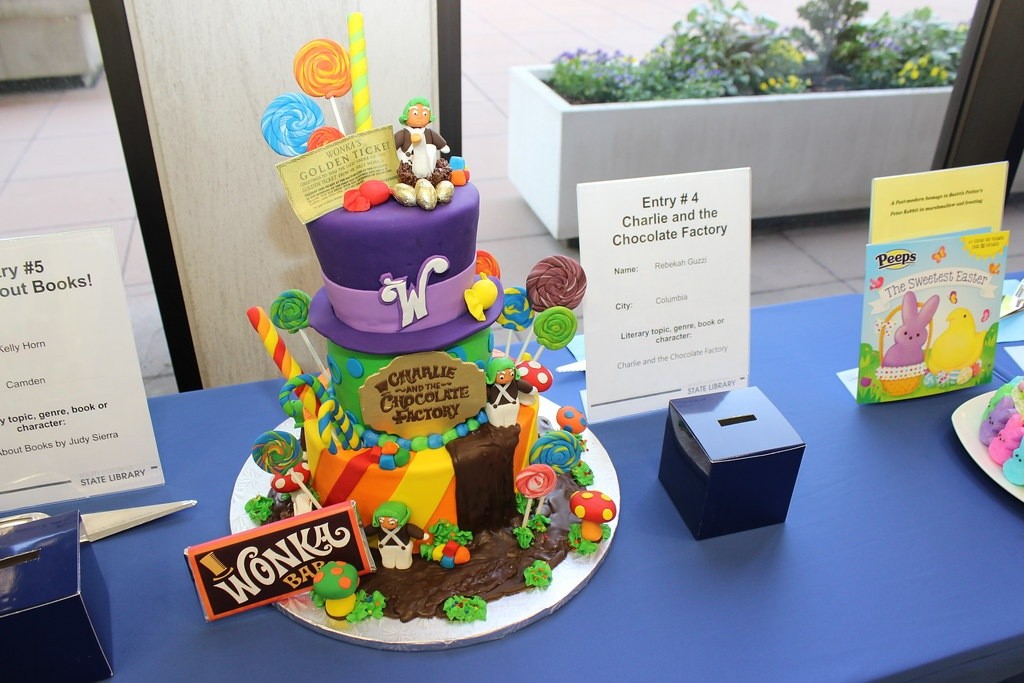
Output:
[244,9,617,621]
[979,374,1024,487]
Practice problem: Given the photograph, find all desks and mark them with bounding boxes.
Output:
[1,272,1023,682]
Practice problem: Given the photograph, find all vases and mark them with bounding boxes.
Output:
[509,61,1024,257]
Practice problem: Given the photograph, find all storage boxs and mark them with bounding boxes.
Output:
[0,510,114,683]
[658,386,805,541]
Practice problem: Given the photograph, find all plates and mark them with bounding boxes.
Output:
[951,391,1024,504]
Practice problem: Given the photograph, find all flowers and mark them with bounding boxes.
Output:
[548,0,970,109]
